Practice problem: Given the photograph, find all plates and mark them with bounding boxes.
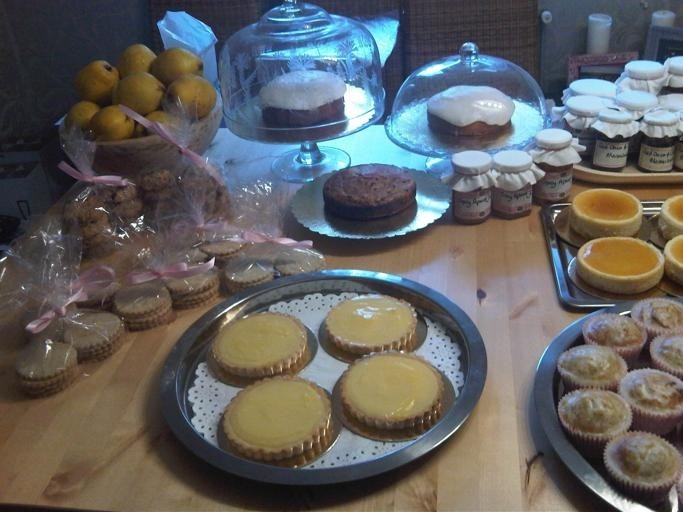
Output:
[154,266,489,490]
[529,297,682,512]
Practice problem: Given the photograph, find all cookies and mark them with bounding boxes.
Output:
[15,241,324,399]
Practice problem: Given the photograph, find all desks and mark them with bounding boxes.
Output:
[0,103,681,511]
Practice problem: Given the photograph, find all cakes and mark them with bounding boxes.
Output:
[211,294,444,463]
[559,301,683,502]
[259,70,346,128]
[568,188,683,295]
[426,85,515,136]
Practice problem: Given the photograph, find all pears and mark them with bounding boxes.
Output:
[66,44,217,142]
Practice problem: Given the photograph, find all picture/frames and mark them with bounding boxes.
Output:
[643,25,682,62]
[567,51,638,93]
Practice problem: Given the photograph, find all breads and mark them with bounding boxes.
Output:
[63,170,228,258]
[323,164,416,221]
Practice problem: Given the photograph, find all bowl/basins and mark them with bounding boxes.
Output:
[59,87,224,181]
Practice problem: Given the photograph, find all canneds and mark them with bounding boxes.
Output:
[451,56,683,225]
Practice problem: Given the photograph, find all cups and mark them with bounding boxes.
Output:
[650,9,675,26]
[585,12,613,55]
